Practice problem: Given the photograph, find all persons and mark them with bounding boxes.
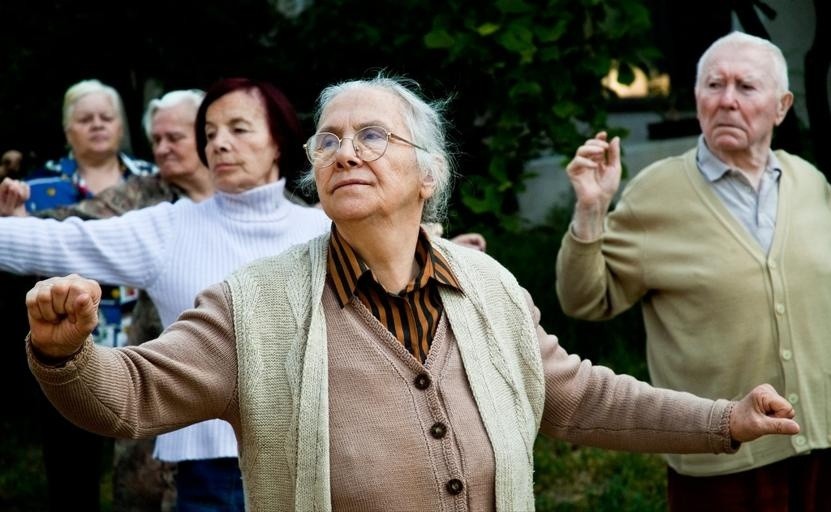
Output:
[1,90,224,511]
[23,66,799,510]
[554,31,830,508]
[0,72,491,512]
[0,79,163,512]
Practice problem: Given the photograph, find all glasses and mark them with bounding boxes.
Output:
[303,126,430,169]
